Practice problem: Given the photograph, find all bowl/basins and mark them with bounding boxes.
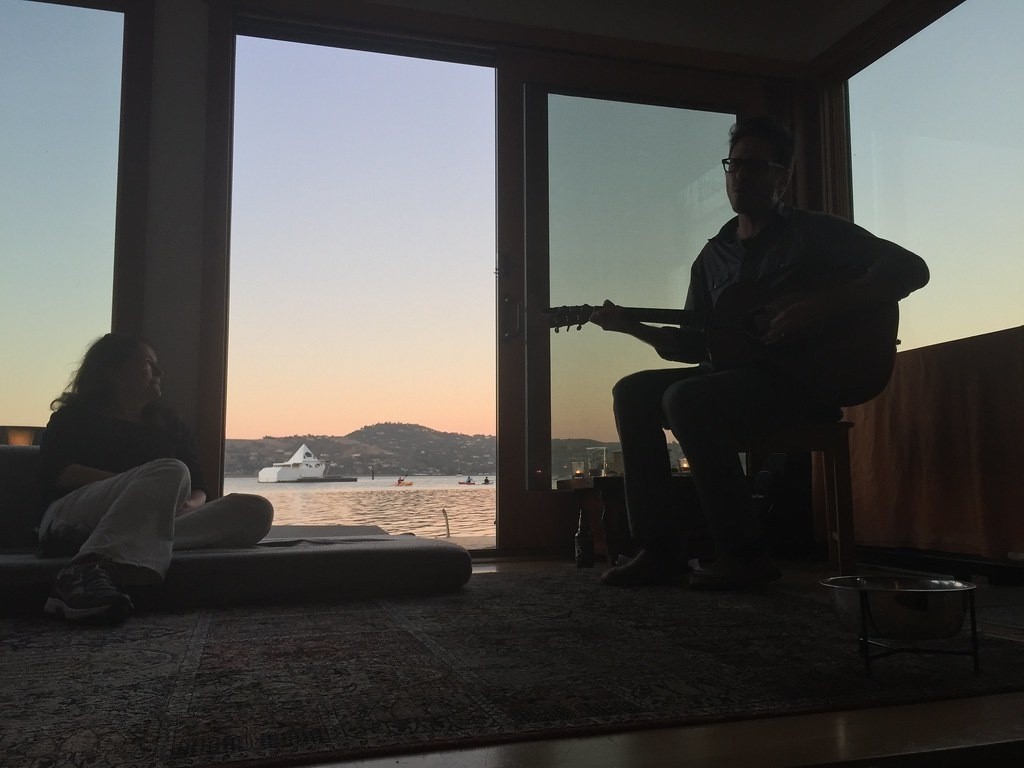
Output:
[823,574,978,642]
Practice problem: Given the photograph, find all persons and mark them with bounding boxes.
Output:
[592,116,929,587]
[38,332,274,620]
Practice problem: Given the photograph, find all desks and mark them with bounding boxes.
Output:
[557,476,715,567]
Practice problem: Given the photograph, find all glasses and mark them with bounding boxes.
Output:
[723,156,784,175]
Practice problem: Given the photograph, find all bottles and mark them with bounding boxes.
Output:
[575,507,595,568]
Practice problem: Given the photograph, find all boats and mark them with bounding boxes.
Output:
[459,482,476,485]
[392,481,414,487]
[256,443,358,483]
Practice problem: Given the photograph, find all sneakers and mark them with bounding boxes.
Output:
[46,559,133,623]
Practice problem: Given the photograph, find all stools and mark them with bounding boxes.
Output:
[739,421,855,575]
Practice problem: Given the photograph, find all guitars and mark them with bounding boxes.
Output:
[549,292,898,408]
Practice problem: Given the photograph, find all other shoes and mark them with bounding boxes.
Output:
[692,556,780,589]
[601,553,690,588]
